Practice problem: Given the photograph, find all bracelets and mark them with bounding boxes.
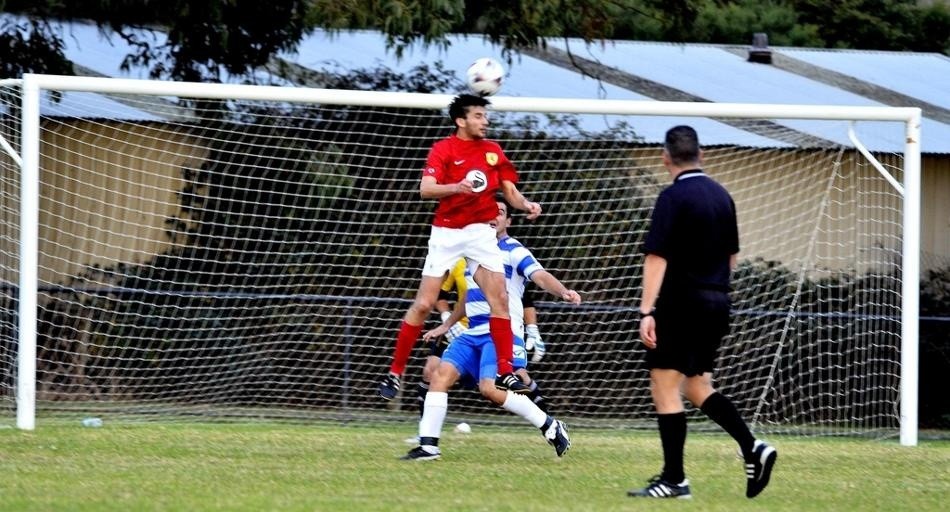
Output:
[636,308,656,320]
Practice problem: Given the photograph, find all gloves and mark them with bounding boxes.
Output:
[441,310,468,343]
[525,322,546,363]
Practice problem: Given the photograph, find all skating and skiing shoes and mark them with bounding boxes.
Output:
[494,371,531,395]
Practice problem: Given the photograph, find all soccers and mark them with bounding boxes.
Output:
[465,58,506,96]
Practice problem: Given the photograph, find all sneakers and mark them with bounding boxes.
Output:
[399,445,442,461]
[379,373,401,403]
[624,477,696,500]
[541,417,571,458]
[736,436,778,499]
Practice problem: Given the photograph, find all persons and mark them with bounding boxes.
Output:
[417,258,547,421]
[624,122,778,499]
[395,193,584,462]
[376,91,535,403]
